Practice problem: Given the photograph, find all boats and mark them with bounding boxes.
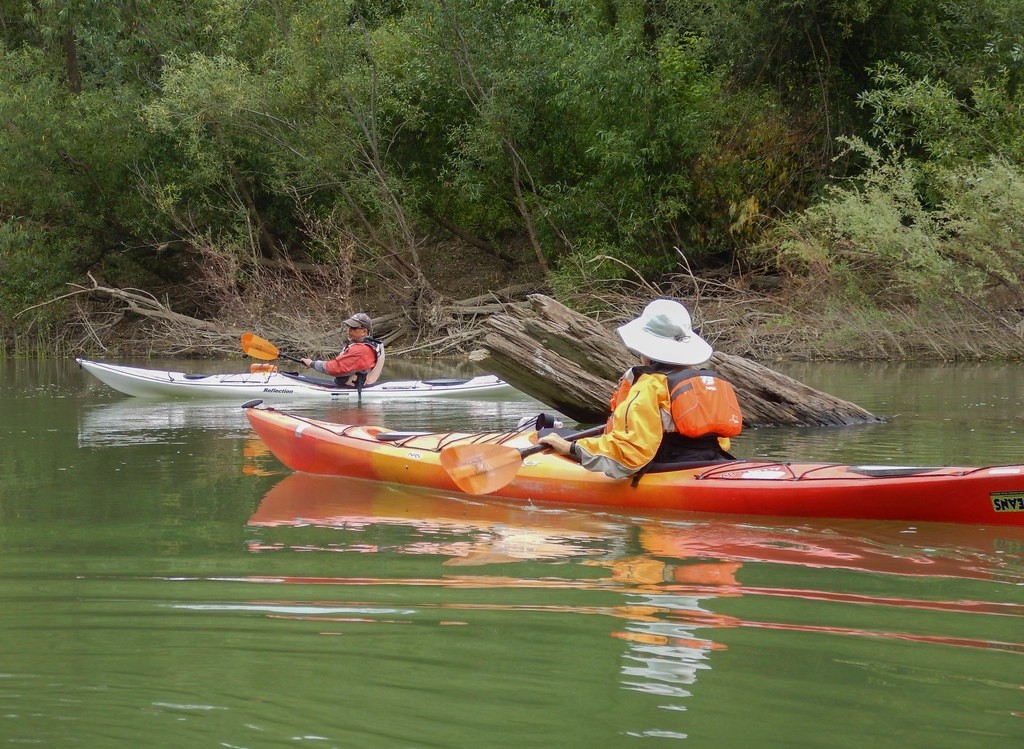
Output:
[74,356,521,401]
[241,398,1024,531]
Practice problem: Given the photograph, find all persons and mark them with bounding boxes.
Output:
[299,313,385,387]
[538,299,743,479]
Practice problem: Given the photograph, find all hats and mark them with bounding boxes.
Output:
[343,313,372,331]
[618,299,713,366]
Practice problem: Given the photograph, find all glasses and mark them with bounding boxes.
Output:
[346,324,363,330]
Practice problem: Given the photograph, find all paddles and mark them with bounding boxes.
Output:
[241,333,305,365]
[439,422,605,495]
